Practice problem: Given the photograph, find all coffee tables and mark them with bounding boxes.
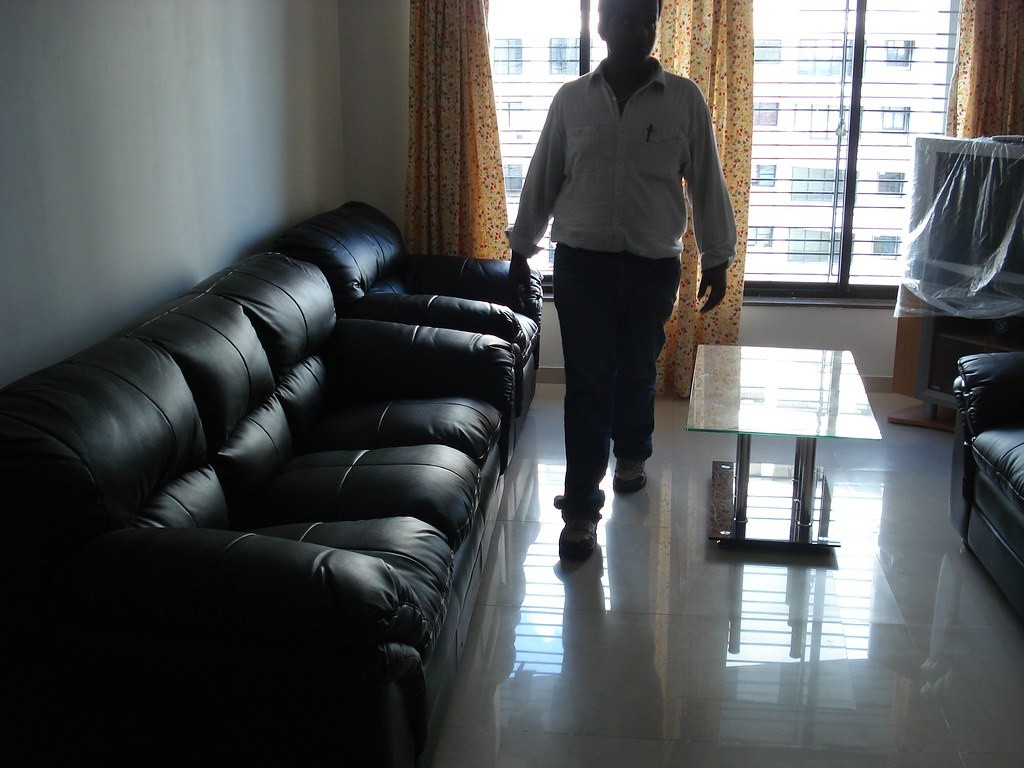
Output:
[683,344,881,547]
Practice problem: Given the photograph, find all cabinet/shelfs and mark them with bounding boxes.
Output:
[912,286,1024,422]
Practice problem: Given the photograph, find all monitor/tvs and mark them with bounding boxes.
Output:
[915,138,1024,307]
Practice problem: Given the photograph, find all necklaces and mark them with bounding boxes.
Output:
[618,59,654,102]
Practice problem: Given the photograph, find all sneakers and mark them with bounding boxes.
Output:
[559,519,597,561]
[613,455,647,493]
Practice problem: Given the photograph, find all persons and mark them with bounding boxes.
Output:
[505,0,737,560]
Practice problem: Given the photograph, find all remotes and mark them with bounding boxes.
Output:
[991,135,1024,143]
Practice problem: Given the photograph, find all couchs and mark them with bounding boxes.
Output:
[950,351,1024,625]
[271,200,543,473]
[0,249,515,768]
